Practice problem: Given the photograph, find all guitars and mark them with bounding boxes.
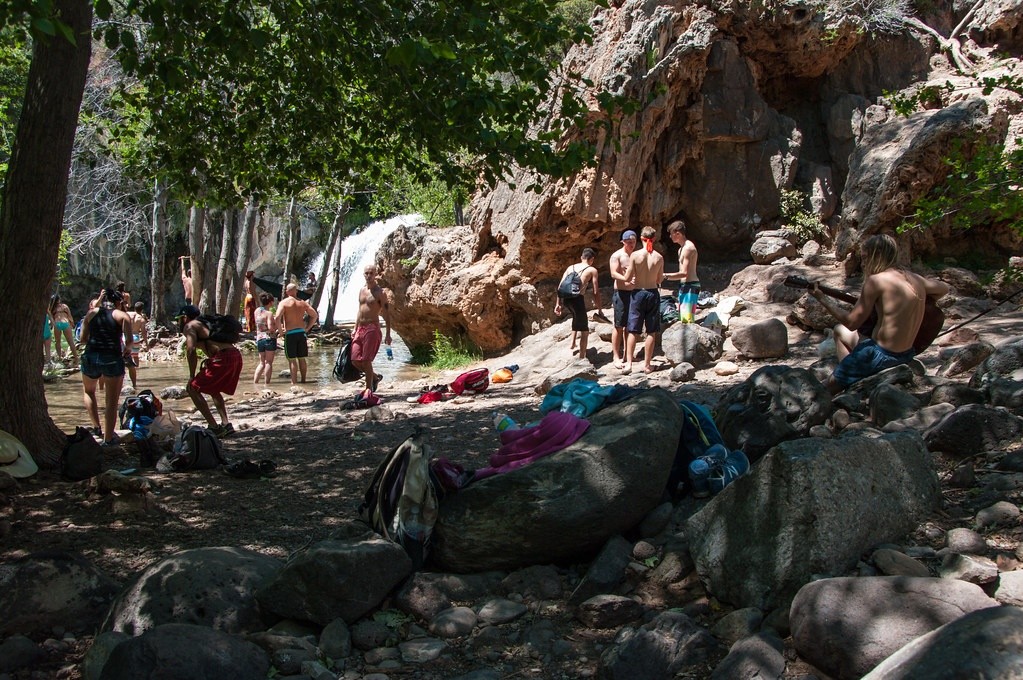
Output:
[784,273,945,356]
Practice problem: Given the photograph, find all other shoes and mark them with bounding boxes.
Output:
[407,386,429,403]
[93,427,101,436]
[103,433,120,446]
[593,313,612,324]
[430,384,449,394]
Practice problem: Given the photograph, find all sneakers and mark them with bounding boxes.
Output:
[688,444,728,498]
[219,422,234,435]
[707,449,749,494]
[206,424,226,439]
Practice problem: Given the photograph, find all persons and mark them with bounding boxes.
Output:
[175,305,243,437]
[554,248,602,358]
[181,255,192,305]
[275,283,317,383]
[253,293,277,384]
[610,229,637,369]
[291,272,318,295]
[663,221,700,323]
[51,294,78,360]
[43,303,54,356]
[621,226,663,373]
[79,281,150,444]
[454,201,463,226]
[810,234,948,395]
[351,264,392,401]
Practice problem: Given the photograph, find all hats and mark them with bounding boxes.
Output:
[620,230,637,242]
[0,430,38,478]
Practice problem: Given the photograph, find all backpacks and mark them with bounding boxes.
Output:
[358,425,447,573]
[195,313,242,357]
[173,426,229,471]
[332,338,365,384]
[557,264,592,299]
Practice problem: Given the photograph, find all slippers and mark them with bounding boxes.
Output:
[372,374,383,392]
[258,459,277,478]
[224,461,261,478]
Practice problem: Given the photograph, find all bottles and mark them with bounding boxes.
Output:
[385,340,393,361]
[491,411,520,432]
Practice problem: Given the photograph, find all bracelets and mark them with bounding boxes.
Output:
[664,275,668,280]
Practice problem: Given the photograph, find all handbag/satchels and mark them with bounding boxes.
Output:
[57,426,102,482]
[671,399,732,481]
